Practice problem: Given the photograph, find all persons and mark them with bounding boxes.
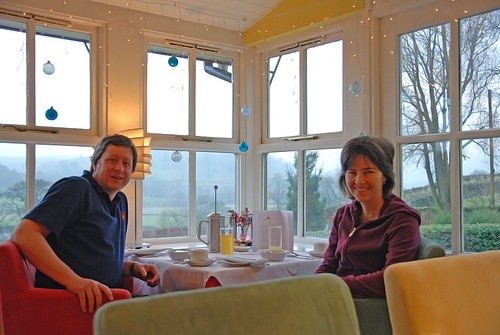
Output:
[11,134,162,313]
[314,135,422,298]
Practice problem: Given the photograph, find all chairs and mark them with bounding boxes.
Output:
[354,236,446,335]
[92,273,360,335]
[381,250,500,335]
[0,238,131,335]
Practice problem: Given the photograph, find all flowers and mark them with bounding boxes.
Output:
[228,207,253,227]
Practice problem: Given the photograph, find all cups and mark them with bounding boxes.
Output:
[268,225,283,250]
[313,241,329,253]
[219,227,233,255]
[190,249,209,262]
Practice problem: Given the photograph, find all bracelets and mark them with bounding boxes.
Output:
[130,262,137,277]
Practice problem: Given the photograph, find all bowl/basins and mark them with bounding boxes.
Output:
[260,248,288,261]
[167,250,190,262]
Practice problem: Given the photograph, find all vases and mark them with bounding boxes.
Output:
[239,225,250,240]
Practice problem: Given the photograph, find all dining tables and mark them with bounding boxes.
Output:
[125,250,325,296]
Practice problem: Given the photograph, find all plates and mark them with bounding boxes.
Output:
[186,254,258,267]
[233,246,250,251]
[133,247,162,257]
[308,250,326,258]
